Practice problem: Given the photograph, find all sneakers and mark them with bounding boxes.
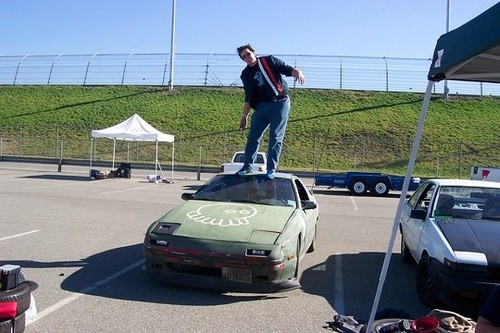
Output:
[266,168,275,179]
[236,165,253,176]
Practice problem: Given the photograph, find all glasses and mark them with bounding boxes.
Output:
[242,52,251,60]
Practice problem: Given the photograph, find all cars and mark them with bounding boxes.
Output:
[398,178,500,289]
[143,173,319,287]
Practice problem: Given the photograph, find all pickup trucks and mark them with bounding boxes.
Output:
[219,151,268,174]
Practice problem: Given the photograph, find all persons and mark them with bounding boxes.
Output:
[235,44,305,180]
[474,283,500,333]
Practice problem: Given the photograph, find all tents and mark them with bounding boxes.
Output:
[89,113,175,184]
[364,1,500,333]
